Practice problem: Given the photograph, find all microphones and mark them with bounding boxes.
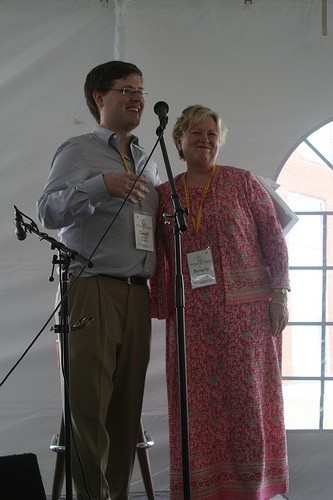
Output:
[153,101,169,129]
[13,211,26,240]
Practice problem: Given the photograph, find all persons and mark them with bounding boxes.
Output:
[36,60,164,500]
[144,103,293,500]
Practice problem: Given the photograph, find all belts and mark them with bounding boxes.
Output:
[100,275,147,285]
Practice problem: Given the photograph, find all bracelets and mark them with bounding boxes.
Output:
[271,299,288,306]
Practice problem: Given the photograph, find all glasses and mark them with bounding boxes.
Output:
[108,86,148,96]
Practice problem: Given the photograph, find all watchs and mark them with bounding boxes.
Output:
[272,288,289,295]
